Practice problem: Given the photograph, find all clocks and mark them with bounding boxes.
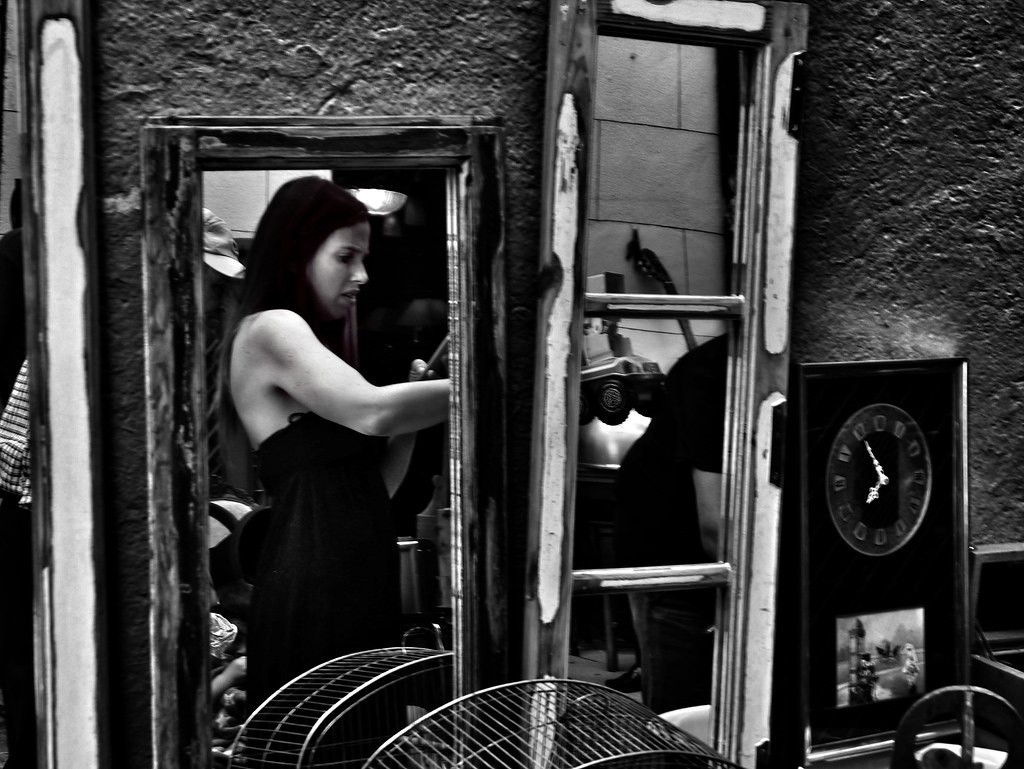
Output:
[792,358,986,769]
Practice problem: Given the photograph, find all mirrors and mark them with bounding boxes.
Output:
[137,113,510,769]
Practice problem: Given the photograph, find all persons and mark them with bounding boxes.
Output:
[214,171,451,769]
[605,655,641,694]
[200,205,271,769]
[606,327,729,720]
